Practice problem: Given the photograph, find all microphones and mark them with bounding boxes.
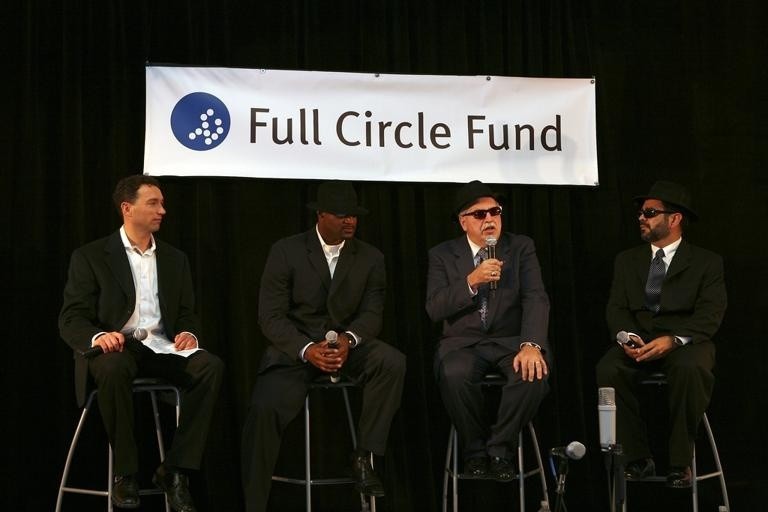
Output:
[550,442,586,460]
[616,331,641,349]
[597,387,617,451]
[326,330,340,383]
[82,328,148,359]
[485,235,497,290]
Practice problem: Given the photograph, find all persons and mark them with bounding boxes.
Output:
[424,179,551,483]
[58,175,224,511]
[595,181,728,489]
[242,180,408,512]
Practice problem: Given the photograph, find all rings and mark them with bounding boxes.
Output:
[491,271,497,276]
[535,361,541,364]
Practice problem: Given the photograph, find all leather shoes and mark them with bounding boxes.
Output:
[152,462,196,512]
[624,458,655,479]
[112,475,140,508]
[465,455,515,482]
[667,466,691,488]
[354,457,384,496]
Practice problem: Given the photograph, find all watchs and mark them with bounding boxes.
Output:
[672,335,683,346]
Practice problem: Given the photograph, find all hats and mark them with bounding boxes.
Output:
[632,180,698,221]
[307,182,370,216]
[451,181,505,223]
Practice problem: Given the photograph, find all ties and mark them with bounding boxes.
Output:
[645,249,665,313]
[476,248,488,325]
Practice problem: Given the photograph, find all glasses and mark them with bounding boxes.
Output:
[638,208,676,218]
[463,207,500,219]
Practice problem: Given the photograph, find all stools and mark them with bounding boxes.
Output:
[611,410,735,512]
[270,381,379,511]
[443,378,553,512]
[54,378,181,511]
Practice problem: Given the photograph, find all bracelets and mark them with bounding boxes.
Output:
[341,331,357,349]
